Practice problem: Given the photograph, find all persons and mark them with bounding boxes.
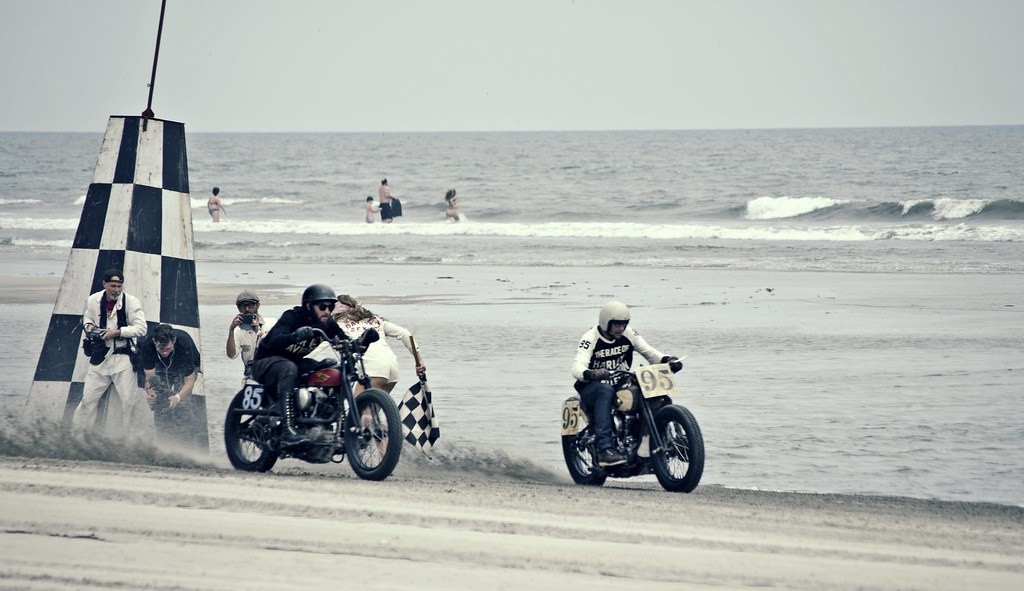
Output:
[71,268,156,443]
[330,293,426,461]
[138,323,201,445]
[253,283,380,448]
[225,289,276,369]
[361,196,378,223]
[378,178,396,223]
[569,301,683,467]
[445,189,459,221]
[207,187,225,221]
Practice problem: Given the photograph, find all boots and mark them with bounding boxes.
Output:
[280,393,310,447]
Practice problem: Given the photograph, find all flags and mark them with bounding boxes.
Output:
[396,379,440,457]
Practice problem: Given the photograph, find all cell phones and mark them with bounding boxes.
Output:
[239,314,253,325]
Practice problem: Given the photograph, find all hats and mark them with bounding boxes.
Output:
[104,268,124,283]
[236,290,259,306]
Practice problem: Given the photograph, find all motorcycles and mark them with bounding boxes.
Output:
[221,328,402,482]
[562,356,706,493]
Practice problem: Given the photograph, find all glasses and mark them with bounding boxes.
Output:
[314,303,335,311]
[611,323,626,328]
[238,303,257,309]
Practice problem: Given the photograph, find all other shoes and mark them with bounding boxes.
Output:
[598,448,627,466]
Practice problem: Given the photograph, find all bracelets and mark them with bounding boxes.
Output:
[176,394,180,402]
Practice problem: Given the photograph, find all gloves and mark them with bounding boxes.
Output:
[361,328,380,344]
[591,368,609,380]
[668,356,682,373]
[296,326,314,340]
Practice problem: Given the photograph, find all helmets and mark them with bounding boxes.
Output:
[599,301,630,332]
[302,284,338,303]
